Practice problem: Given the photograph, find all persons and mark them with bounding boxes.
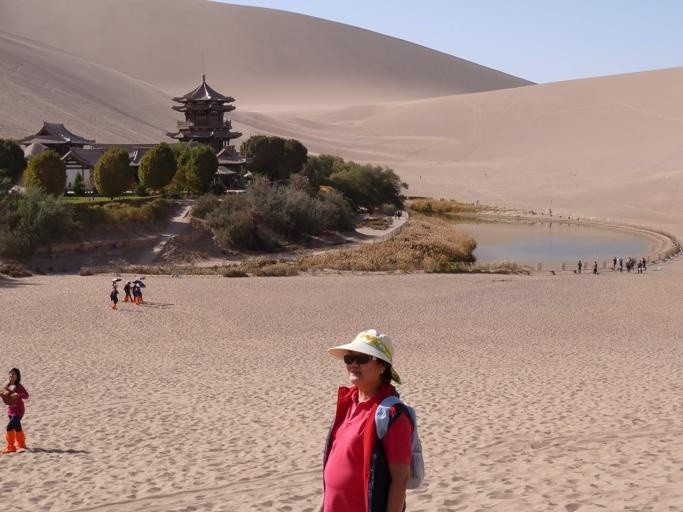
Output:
[109,281,143,309]
[576,256,648,276]
[316,328,415,512]
[0,367,29,455]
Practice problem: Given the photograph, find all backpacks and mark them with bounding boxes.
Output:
[0,387,16,406]
[374,395,426,490]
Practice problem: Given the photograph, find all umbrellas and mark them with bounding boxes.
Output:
[111,278,121,282]
[134,275,146,280]
[133,281,145,288]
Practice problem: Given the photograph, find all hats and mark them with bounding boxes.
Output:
[326,328,403,385]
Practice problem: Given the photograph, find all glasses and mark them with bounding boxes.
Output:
[343,353,379,365]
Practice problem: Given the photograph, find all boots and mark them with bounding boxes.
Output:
[15,431,27,450]
[2,430,17,453]
